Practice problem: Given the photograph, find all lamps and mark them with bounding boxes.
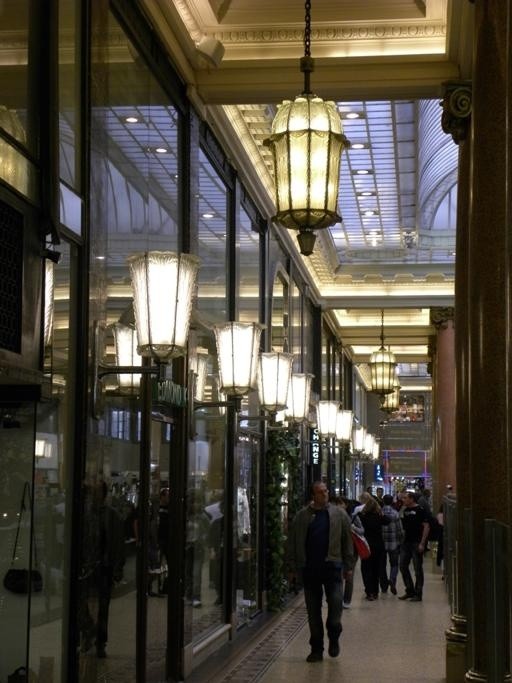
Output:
[365,308,398,404]
[379,379,402,415]
[262,0,351,256]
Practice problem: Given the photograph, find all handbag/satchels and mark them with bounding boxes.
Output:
[3,565,45,594]
[349,515,372,562]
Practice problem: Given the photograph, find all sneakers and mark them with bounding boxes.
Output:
[306,651,324,662]
[341,599,353,609]
[327,636,340,657]
[78,621,99,654]
[96,642,108,660]
[185,594,222,609]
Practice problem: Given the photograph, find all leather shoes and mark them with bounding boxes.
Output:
[388,578,398,594]
[399,591,415,600]
[408,595,422,602]
[365,592,378,601]
[381,588,387,593]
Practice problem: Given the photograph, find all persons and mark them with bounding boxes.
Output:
[76,480,223,659]
[332,486,444,609]
[289,480,354,663]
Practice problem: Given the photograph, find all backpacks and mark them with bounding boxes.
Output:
[416,508,443,541]
